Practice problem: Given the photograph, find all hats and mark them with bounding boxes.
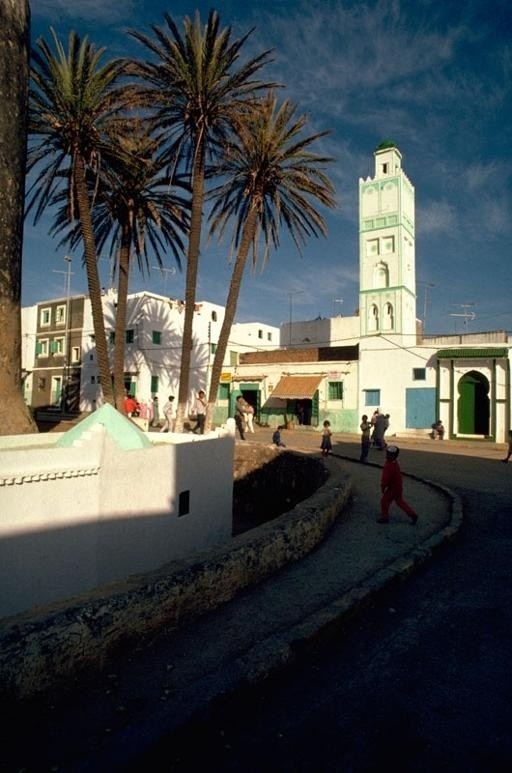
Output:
[386,446,399,454]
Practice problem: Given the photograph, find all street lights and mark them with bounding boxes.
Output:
[60,253,72,416]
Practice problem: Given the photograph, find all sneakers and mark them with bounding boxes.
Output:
[377,518,389,524]
[408,514,418,525]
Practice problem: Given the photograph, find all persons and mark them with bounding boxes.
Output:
[158,395,176,432]
[501,429,512,463]
[296,403,304,425]
[234,395,247,440]
[271,425,286,448]
[191,390,209,434]
[370,410,390,451]
[240,397,249,423]
[432,419,444,440]
[320,420,333,457]
[124,389,162,427]
[358,414,373,463]
[375,444,419,527]
[240,399,255,433]
